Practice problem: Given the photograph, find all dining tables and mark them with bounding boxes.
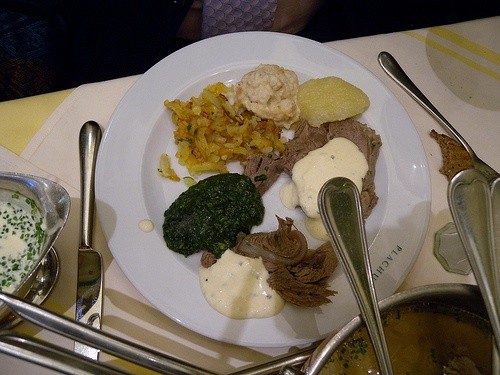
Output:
[0,14,500,375]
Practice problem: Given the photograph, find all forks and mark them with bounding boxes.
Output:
[379,52,500,184]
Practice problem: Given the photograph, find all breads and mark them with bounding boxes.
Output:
[430,129,474,181]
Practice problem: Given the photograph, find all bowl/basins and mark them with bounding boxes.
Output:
[0,172,71,313]
[299,282,500,375]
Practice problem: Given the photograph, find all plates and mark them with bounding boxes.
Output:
[93,31,431,348]
[0,245,60,330]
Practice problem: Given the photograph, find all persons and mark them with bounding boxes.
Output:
[176,0,318,42]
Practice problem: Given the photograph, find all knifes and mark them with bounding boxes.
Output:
[70,120,102,363]
